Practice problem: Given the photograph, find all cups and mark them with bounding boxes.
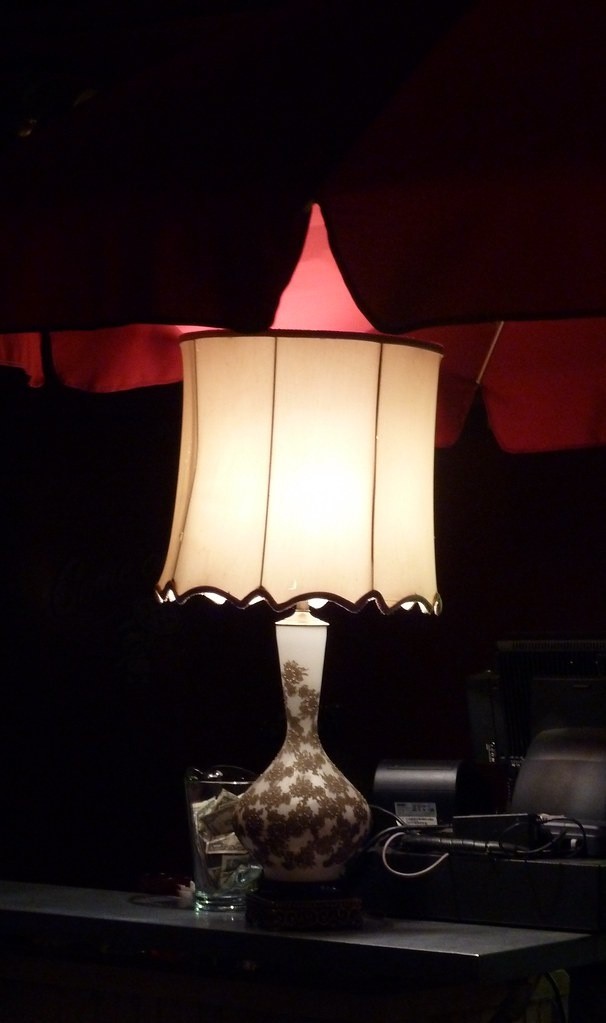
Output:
[186,772,253,922]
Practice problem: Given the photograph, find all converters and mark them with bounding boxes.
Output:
[452,812,540,850]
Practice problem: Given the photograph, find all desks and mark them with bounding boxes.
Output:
[0,881,591,1023]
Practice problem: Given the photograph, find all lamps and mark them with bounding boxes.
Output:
[149,329,446,887]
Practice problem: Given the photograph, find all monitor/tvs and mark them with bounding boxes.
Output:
[495,637,605,755]
